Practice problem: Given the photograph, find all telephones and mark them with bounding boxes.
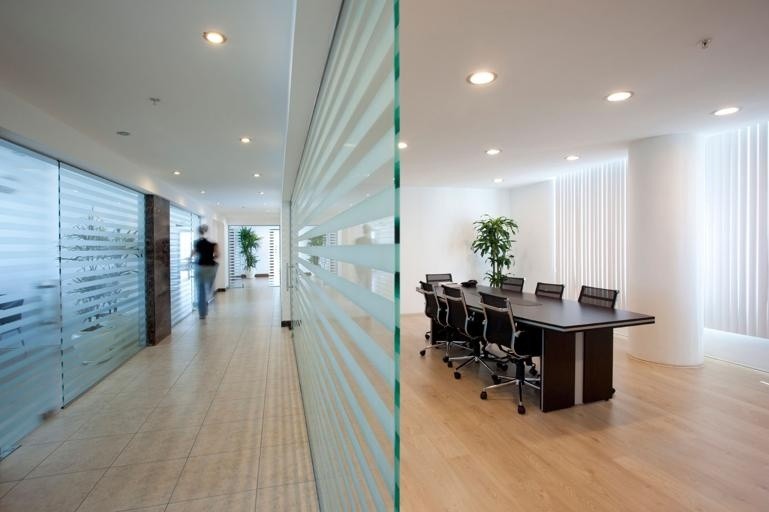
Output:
[461,280,477,287]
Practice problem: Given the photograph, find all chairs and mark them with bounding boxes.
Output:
[0,298,26,348]
[416,273,657,415]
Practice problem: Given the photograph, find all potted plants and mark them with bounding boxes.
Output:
[60,208,142,366]
[238,225,261,279]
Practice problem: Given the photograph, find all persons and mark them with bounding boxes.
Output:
[189,224,221,319]
[352,222,375,292]
